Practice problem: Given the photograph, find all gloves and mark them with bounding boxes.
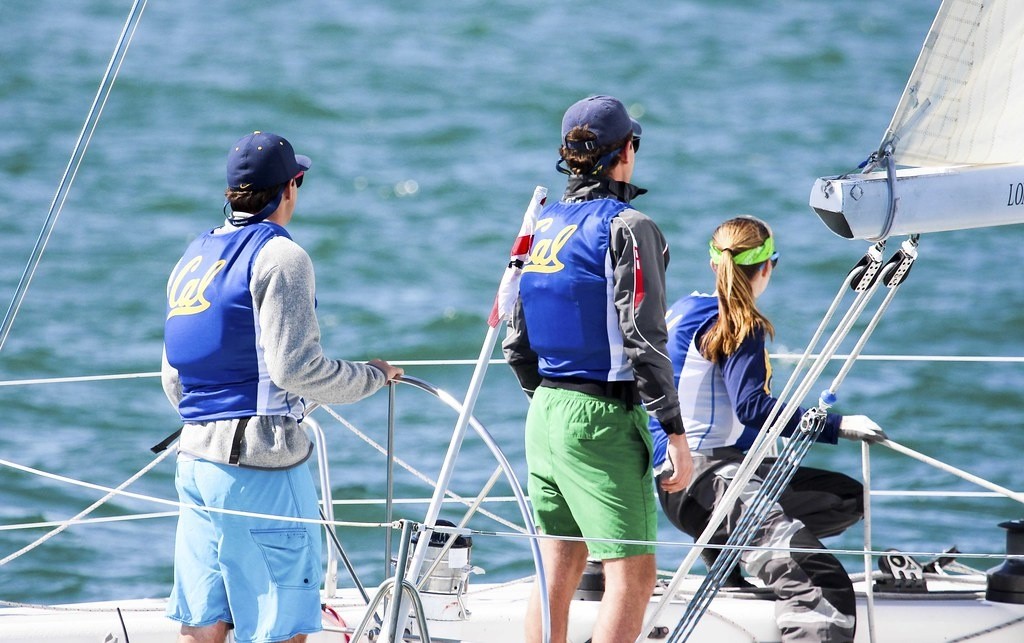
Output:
[838,414,887,446]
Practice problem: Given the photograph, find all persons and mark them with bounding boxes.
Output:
[162,131,404,642]
[656,215,887,643]
[502,96,693,643]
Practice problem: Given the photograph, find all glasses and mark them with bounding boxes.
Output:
[759,251,779,271]
[631,136,641,153]
[295,175,303,188]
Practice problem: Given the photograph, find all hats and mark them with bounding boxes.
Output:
[561,95,642,151]
[227,131,312,192]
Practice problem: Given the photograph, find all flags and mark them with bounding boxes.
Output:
[488,190,546,327]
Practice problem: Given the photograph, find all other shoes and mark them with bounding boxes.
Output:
[695,534,754,587]
